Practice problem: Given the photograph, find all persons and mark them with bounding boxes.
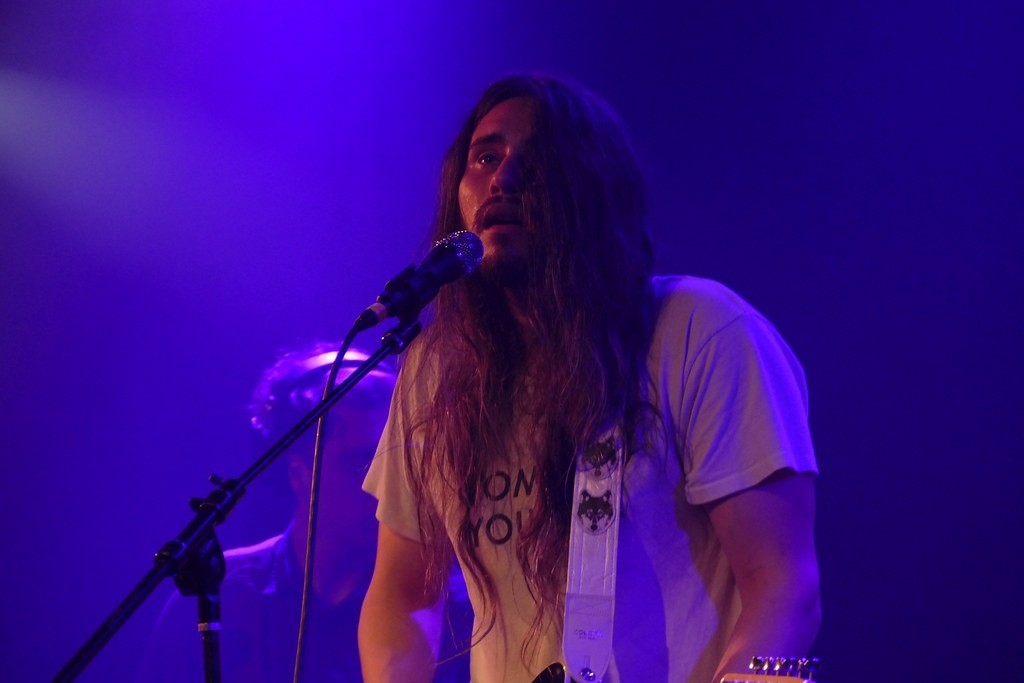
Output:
[355,72,822,682]
[144,342,466,680]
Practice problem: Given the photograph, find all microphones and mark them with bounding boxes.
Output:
[352,230,483,329]
